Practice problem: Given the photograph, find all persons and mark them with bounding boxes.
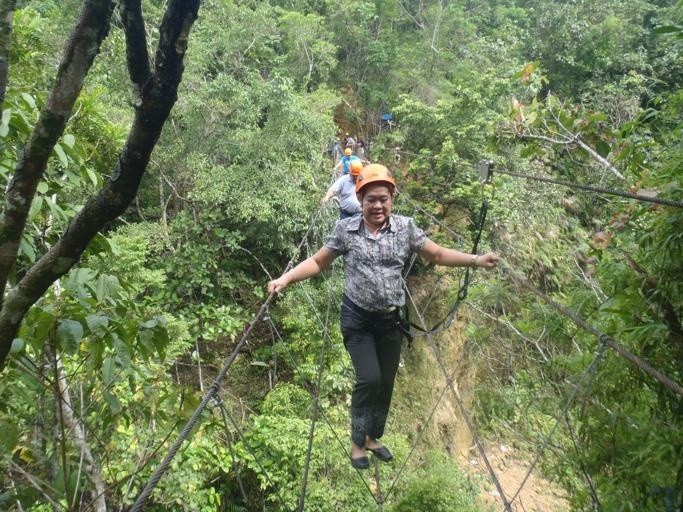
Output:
[266,165,497,467]
[343,132,349,145]
[320,161,363,220]
[345,134,362,154]
[333,147,361,175]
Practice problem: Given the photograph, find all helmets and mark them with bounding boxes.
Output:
[350,160,363,176]
[345,148,352,157]
[355,164,396,193]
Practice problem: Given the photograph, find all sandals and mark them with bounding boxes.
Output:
[364,440,393,461]
[350,449,370,470]
